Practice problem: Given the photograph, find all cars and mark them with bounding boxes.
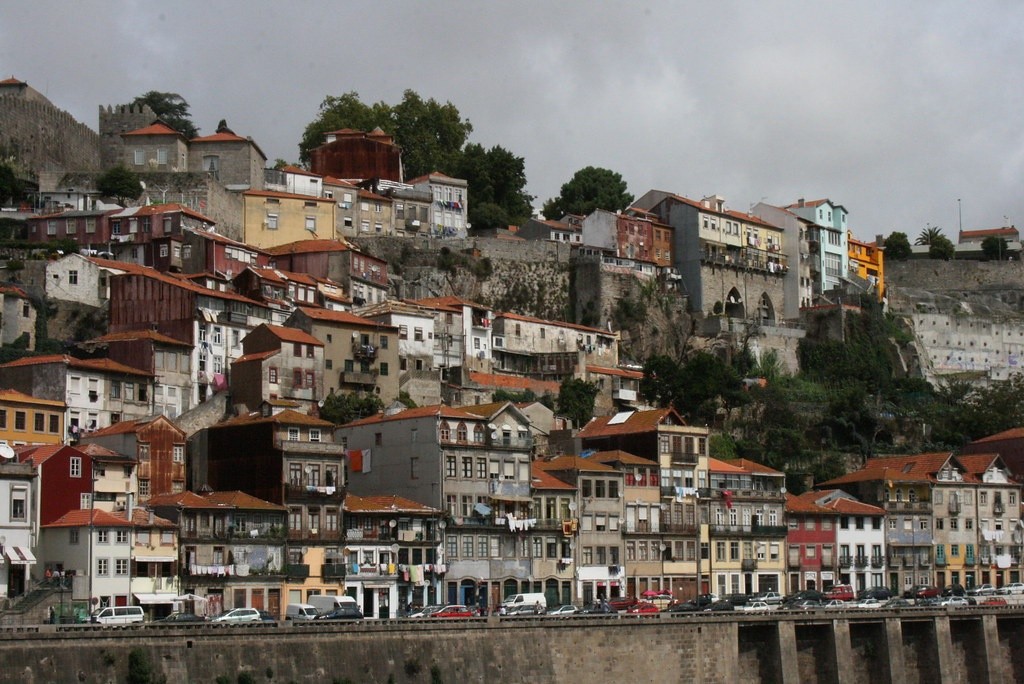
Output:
[547,605,578,621]
[405,606,443,622]
[315,607,363,626]
[425,605,477,624]
[573,602,618,621]
[151,613,205,631]
[607,597,636,610]
[506,605,546,617]
[668,579,1024,618]
[626,602,660,618]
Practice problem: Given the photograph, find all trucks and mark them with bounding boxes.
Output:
[308,594,360,613]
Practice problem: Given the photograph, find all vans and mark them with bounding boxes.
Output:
[285,604,320,626]
[82,606,147,631]
[502,593,548,612]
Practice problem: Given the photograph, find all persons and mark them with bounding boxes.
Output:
[534,601,543,614]
[359,606,363,614]
[50,607,56,623]
[45,568,72,586]
[603,600,610,620]
[600,592,604,604]
[479,605,486,616]
[407,602,412,612]
[201,614,209,621]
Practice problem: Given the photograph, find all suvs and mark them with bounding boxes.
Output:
[208,607,262,629]
